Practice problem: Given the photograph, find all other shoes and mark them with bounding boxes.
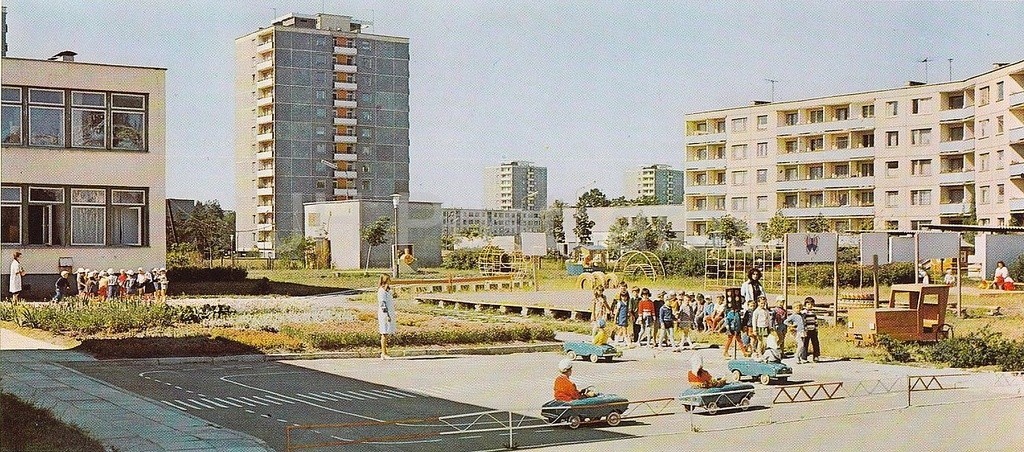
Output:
[381,353,394,359]
[796,357,801,364]
[814,356,819,362]
[801,359,809,363]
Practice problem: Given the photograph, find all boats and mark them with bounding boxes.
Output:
[565,245,608,276]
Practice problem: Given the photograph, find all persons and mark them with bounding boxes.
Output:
[993,261,1014,290]
[591,282,820,364]
[52,271,71,302]
[740,268,765,344]
[943,268,955,284]
[688,356,726,388]
[755,334,782,362]
[377,273,396,359]
[918,264,929,284]
[76,267,170,300]
[9,252,25,303]
[553,359,594,401]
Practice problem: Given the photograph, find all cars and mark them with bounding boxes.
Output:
[564,340,623,363]
[728,358,793,385]
[541,386,629,430]
[679,382,755,414]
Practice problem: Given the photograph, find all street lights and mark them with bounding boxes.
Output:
[229,235,236,280]
[390,194,401,278]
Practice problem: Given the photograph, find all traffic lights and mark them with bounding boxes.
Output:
[726,288,742,309]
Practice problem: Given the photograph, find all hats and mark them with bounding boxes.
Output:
[125,269,135,276]
[120,269,125,272]
[160,267,167,272]
[687,291,694,296]
[77,268,107,278]
[107,269,115,275]
[716,292,723,296]
[61,271,69,276]
[138,267,146,274]
[152,268,159,273]
[776,295,785,300]
[704,295,712,300]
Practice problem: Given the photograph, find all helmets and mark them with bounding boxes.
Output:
[558,358,572,372]
